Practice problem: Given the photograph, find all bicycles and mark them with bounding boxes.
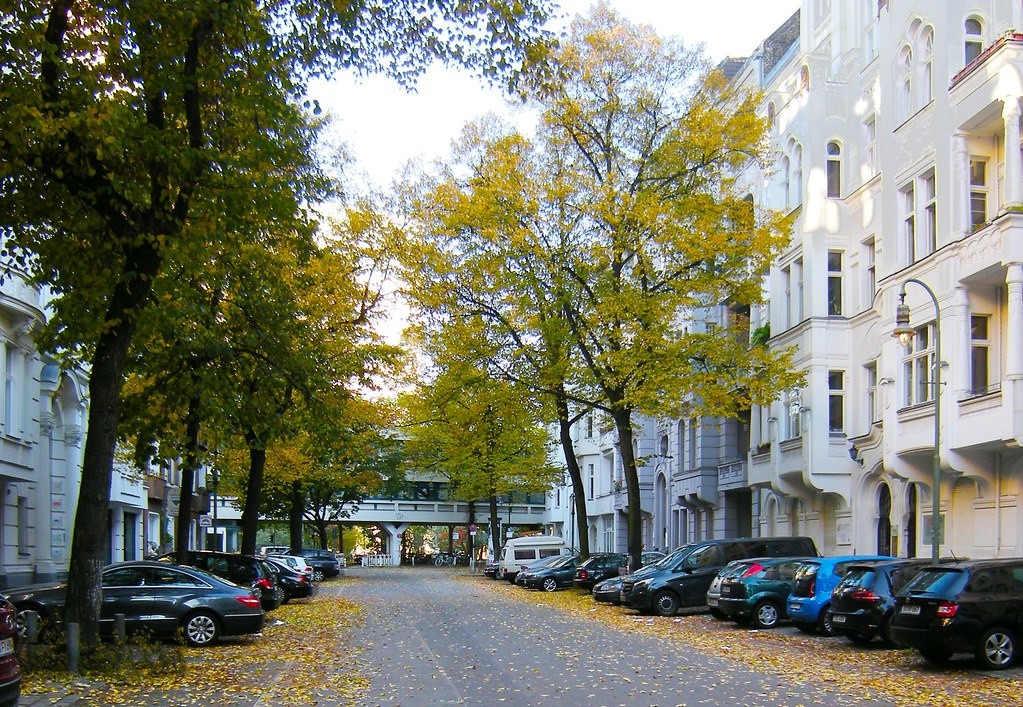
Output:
[434,550,454,566]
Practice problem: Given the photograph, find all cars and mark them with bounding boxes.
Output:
[619,551,668,577]
[253,555,312,604]
[0,595,20,705]
[279,549,339,582]
[827,557,969,650]
[484,562,503,580]
[894,558,1023,669]
[718,558,816,629]
[0,561,266,649]
[706,557,774,620]
[259,555,315,578]
[786,556,902,636]
[514,555,589,592]
[147,550,280,612]
[573,554,625,592]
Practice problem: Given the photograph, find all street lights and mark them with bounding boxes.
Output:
[891,278,939,558]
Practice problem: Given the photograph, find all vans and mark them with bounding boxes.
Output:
[620,537,818,616]
[499,536,580,585]
